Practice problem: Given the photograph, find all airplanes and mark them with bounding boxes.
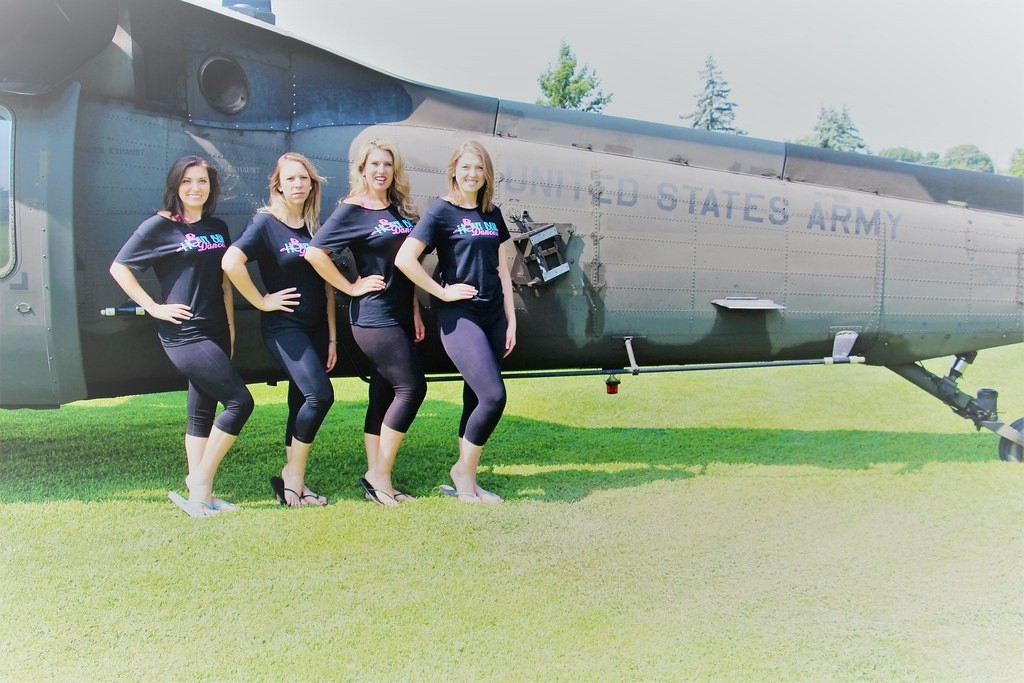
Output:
[0,0,1024,462]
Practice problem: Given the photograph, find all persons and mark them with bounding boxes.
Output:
[393,140,516,506]
[304,134,428,508]
[110,155,254,515]
[220,153,338,508]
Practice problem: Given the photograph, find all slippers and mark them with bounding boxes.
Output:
[167,491,214,517]
[364,491,416,503]
[360,478,396,507]
[474,488,504,504]
[270,476,304,506]
[301,490,328,506]
[213,501,229,510]
[439,485,478,503]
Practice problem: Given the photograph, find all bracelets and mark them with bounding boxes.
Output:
[327,340,337,344]
